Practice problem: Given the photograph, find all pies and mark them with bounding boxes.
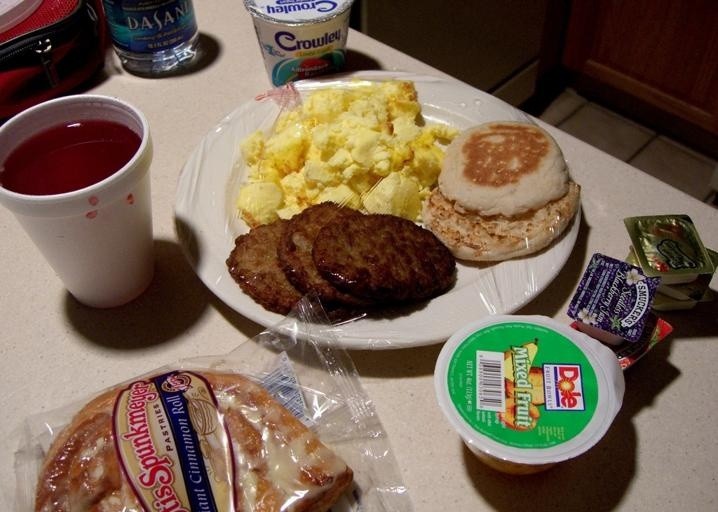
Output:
[227,201,457,324]
[422,120,581,262]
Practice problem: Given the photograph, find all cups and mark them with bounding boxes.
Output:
[244,1,353,88]
[0,90,154,312]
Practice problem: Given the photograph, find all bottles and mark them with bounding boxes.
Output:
[101,1,199,78]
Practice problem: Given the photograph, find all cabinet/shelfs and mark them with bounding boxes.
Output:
[350,0,564,108]
[559,0,718,141]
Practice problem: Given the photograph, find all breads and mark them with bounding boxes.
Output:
[34,368,354,512]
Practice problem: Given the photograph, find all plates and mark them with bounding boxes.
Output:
[172,66,583,352]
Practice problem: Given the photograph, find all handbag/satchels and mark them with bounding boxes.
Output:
[1,2,109,124]
